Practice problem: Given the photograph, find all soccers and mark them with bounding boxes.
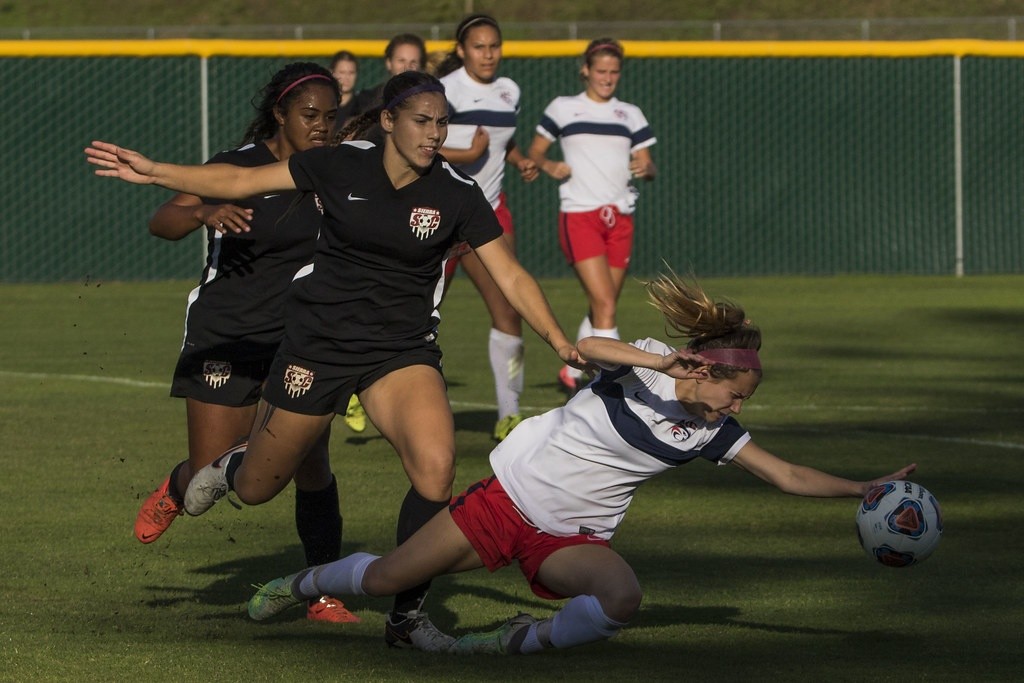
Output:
[852,477,943,573]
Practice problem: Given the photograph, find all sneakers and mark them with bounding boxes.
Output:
[449,614,537,655]
[134,460,184,543]
[493,415,521,440]
[384,612,456,650]
[559,365,578,398]
[184,443,248,517]
[248,565,316,620]
[344,393,366,433]
[307,595,359,622]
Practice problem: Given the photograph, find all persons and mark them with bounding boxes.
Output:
[135,14,658,621]
[84,70,603,652]
[247,281,918,658]
